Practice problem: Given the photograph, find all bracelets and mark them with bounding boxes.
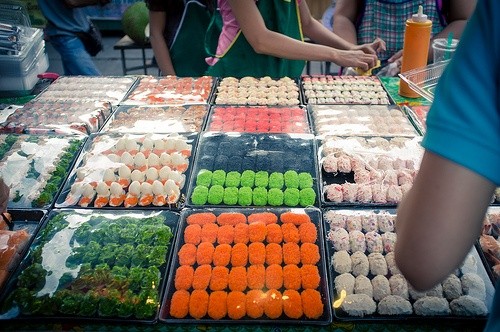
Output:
[160,72,176,76]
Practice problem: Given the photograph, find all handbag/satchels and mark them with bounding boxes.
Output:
[75,24,104,56]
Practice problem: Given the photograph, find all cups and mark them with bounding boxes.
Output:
[432,39,460,64]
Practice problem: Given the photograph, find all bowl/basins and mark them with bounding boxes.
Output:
[397,59,452,103]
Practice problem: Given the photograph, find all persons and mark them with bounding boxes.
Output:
[393,0,500,332]
[0,178,9,214]
[204,0,386,79]
[332,0,478,77]
[144,0,213,77]
[37,0,112,76]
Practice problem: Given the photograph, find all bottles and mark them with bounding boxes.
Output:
[398,5,432,98]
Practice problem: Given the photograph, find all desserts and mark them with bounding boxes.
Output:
[0,75,500,320]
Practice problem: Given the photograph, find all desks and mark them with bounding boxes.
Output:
[112,33,153,75]
